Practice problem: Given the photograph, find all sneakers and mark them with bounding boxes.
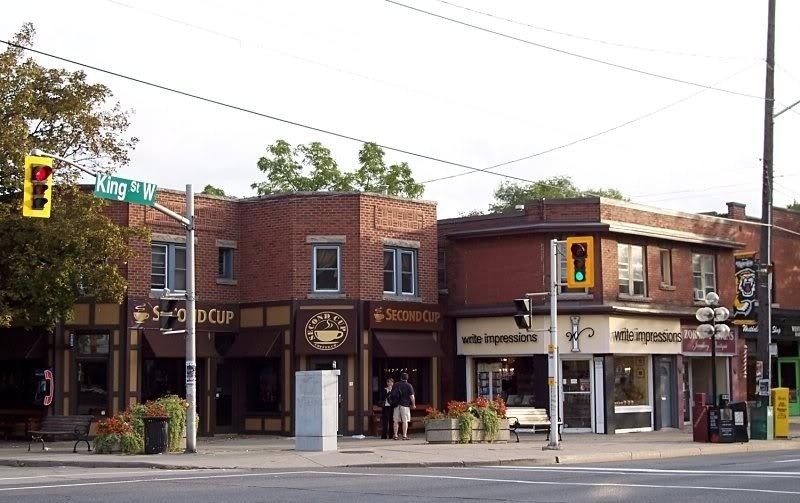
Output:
[394,436,411,440]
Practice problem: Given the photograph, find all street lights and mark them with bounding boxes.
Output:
[695,290,731,410]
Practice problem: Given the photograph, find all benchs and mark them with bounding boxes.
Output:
[28,415,94,453]
[502,407,562,442]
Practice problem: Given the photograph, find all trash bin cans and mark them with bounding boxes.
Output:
[141,416,172,454]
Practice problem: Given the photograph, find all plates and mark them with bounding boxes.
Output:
[314,342,340,345]
[135,321,144,324]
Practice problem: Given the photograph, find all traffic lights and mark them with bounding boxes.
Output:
[512,296,533,329]
[160,295,179,332]
[22,152,52,218]
[567,235,595,288]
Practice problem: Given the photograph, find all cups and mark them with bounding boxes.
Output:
[133,312,149,321]
[313,330,344,342]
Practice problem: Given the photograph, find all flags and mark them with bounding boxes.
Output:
[732,251,758,325]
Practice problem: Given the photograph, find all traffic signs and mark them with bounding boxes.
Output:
[93,172,157,208]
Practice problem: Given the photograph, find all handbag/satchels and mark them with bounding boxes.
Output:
[391,386,402,406]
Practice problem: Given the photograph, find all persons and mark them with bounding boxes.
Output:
[391,372,417,440]
[380,377,395,439]
